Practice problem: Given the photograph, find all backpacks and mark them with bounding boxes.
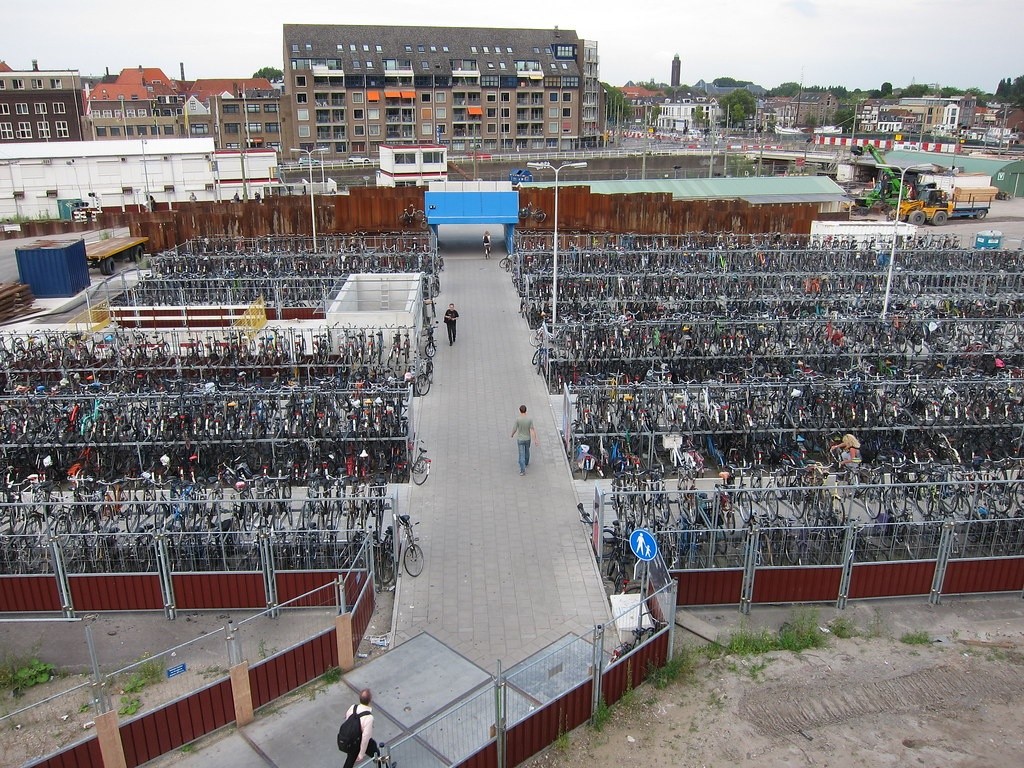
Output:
[851,444,875,463]
[483,236,490,243]
[336,705,372,754]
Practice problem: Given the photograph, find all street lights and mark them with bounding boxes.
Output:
[526,162,588,334]
[290,148,328,253]
[876,162,934,323]
[140,133,152,213]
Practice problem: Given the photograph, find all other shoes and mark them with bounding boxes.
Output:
[453,339,456,342]
[390,762,397,768]
[449,341,452,347]
[520,471,525,476]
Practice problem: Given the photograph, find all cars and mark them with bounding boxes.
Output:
[347,154,371,164]
[299,157,319,166]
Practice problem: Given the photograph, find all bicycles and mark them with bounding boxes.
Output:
[110,230,445,316]
[0,322,438,584]
[399,205,425,226]
[500,228,1024,595]
[485,246,491,260]
[518,202,546,222]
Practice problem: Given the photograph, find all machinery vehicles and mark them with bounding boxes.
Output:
[851,145,909,209]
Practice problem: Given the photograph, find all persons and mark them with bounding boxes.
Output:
[234,192,239,202]
[444,303,459,345]
[254,191,261,200]
[190,192,197,201]
[483,230,491,259]
[511,405,539,475]
[830,433,861,467]
[143,196,157,212]
[342,688,397,768]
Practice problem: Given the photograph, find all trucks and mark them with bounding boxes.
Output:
[886,174,999,227]
